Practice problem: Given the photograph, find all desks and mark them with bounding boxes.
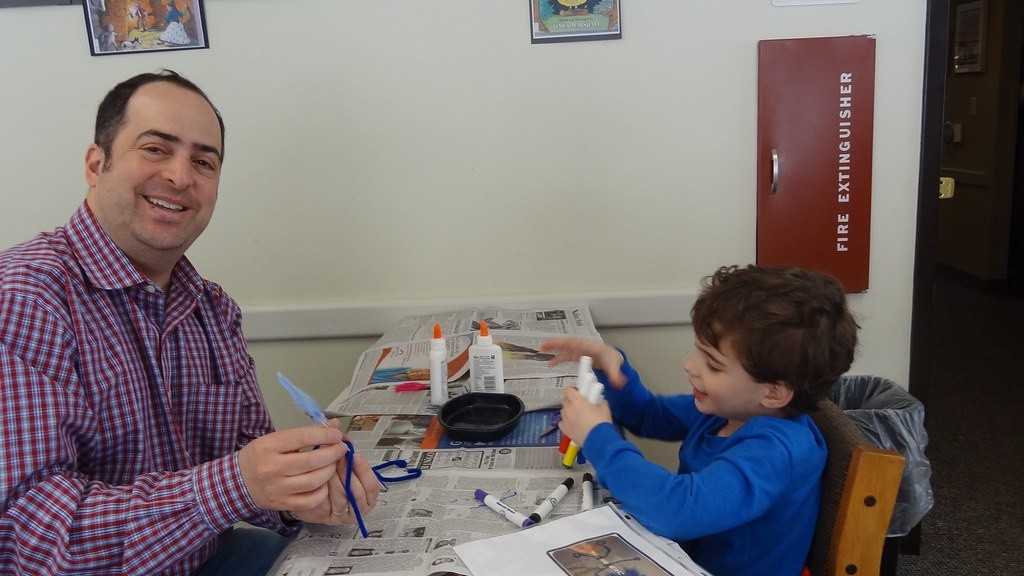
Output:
[261,308,628,576]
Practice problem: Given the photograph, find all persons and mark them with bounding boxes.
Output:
[541,266,862,576]
[0,70,379,576]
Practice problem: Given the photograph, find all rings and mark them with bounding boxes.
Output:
[331,505,349,516]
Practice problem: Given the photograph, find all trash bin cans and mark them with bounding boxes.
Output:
[828,375,920,576]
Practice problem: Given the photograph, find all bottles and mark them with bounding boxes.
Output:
[430,322,448,406]
[469,321,504,393]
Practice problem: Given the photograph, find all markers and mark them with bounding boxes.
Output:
[558,356,605,468]
[474,488,536,528]
[581,472,594,512]
[529,477,574,524]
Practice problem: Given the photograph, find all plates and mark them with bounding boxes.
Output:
[438,392,525,441]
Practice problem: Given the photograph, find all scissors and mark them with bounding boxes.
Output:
[539,411,562,438]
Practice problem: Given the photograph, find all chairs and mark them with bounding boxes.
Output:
[808,395,907,576]
